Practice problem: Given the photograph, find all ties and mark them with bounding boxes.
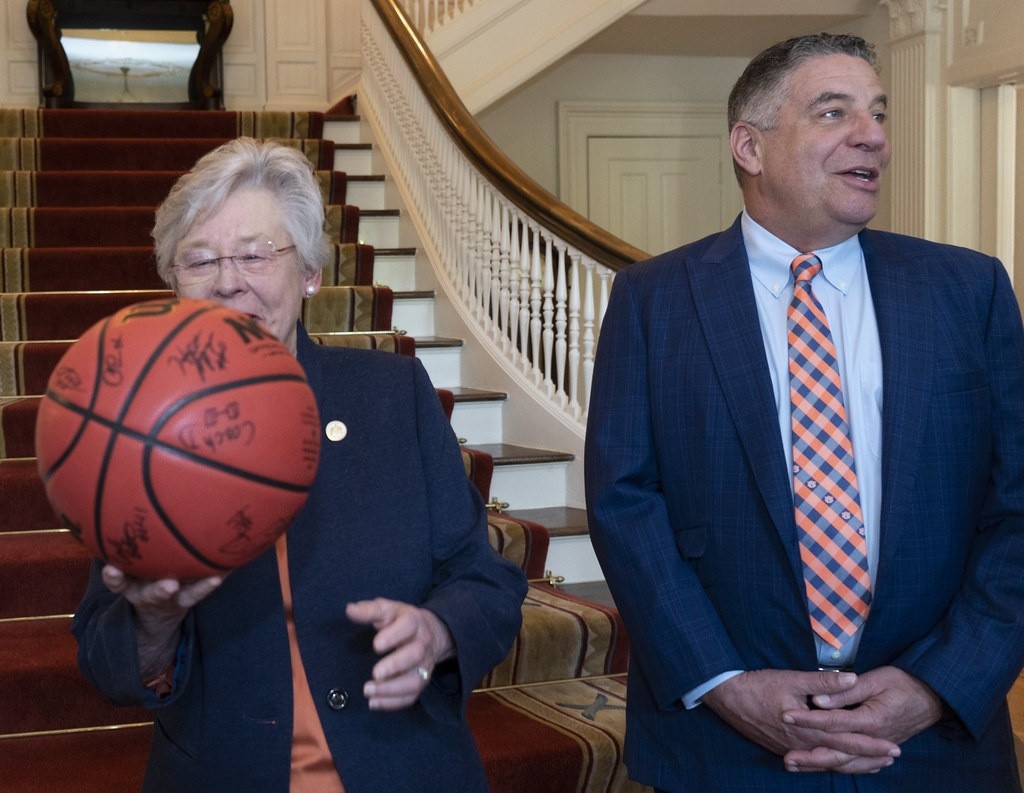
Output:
[786,252,873,650]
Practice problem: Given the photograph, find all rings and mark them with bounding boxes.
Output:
[411,667,427,683]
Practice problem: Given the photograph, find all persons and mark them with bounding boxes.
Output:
[73,137,528,793]
[582,34,1023,793]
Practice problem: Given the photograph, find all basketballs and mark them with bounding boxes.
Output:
[35,298,321,583]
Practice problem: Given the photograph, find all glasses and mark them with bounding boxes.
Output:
[169,240,295,286]
